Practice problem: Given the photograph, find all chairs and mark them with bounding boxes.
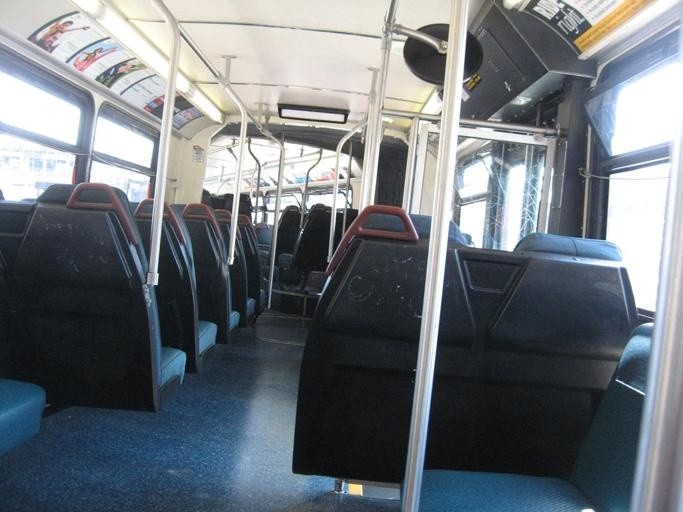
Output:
[2,183,357,511]
[291,203,681,512]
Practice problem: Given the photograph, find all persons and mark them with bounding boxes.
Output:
[34,18,73,51]
[76,45,103,71]
[113,64,136,74]
